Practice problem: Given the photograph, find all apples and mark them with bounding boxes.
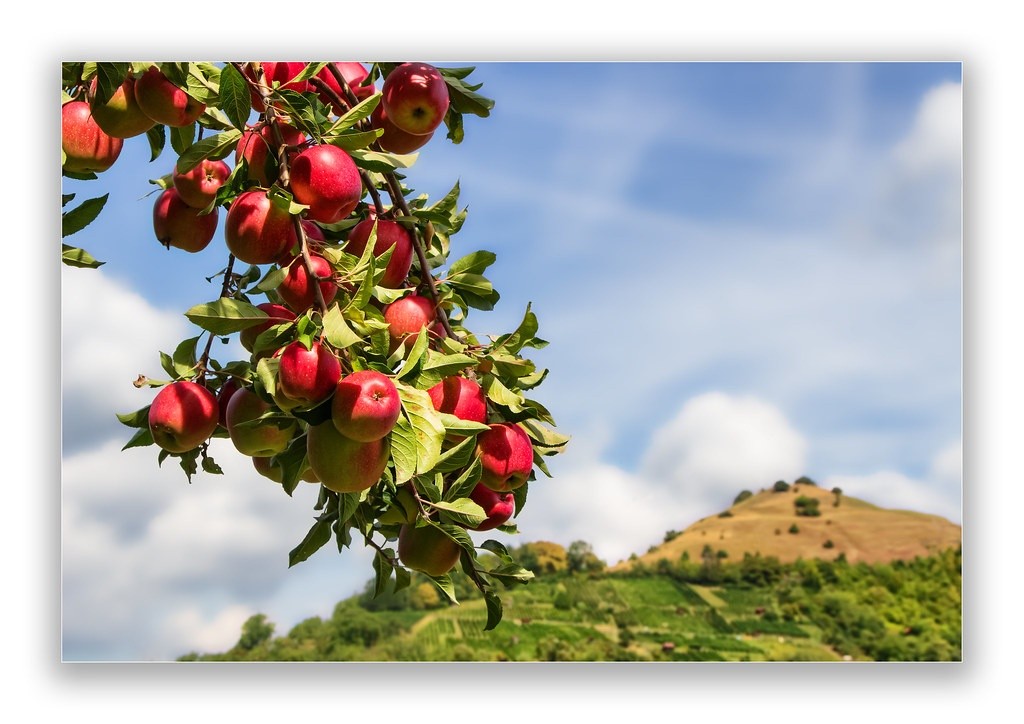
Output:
[62,62,531,576]
